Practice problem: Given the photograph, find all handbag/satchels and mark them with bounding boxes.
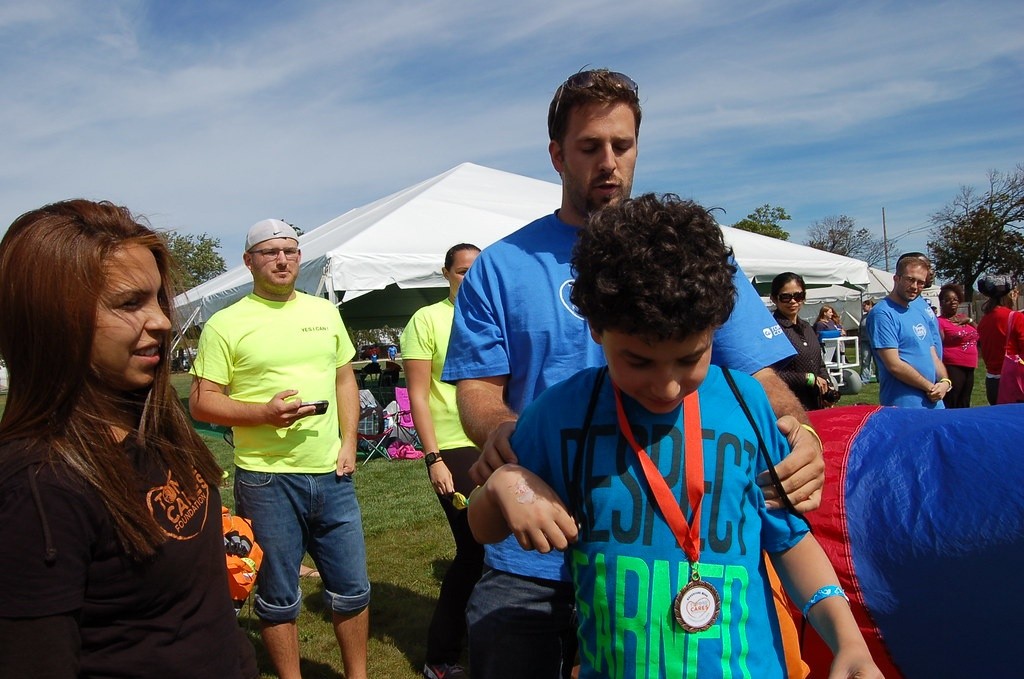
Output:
[997,311,1023,405]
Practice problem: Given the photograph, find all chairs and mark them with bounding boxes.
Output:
[359,388,393,466]
[382,387,424,454]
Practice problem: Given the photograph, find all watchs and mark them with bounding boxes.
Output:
[940,378,952,392]
[425,452,441,466]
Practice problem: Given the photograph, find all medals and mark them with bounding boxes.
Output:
[674,571,720,633]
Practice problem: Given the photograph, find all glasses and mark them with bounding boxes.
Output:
[552,70,639,139]
[248,248,300,260]
[898,275,925,288]
[778,291,806,303]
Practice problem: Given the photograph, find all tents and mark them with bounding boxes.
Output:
[170,161,870,367]
[759,266,942,333]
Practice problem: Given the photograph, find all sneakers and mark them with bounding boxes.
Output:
[424,662,470,679]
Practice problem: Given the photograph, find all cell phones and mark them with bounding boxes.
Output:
[300,401,329,415]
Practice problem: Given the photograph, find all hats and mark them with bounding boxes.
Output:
[245,218,299,251]
[978,275,1017,296]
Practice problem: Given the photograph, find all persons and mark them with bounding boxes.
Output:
[189,219,371,679]
[0,198,260,679]
[866,252,1024,412]
[467,190,886,679]
[439,67,885,679]
[770,272,840,411]
[400,243,482,679]
[813,300,877,386]
[387,344,398,363]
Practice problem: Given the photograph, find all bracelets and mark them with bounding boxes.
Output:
[803,585,849,619]
[968,318,973,324]
[801,423,823,454]
[806,373,814,386]
